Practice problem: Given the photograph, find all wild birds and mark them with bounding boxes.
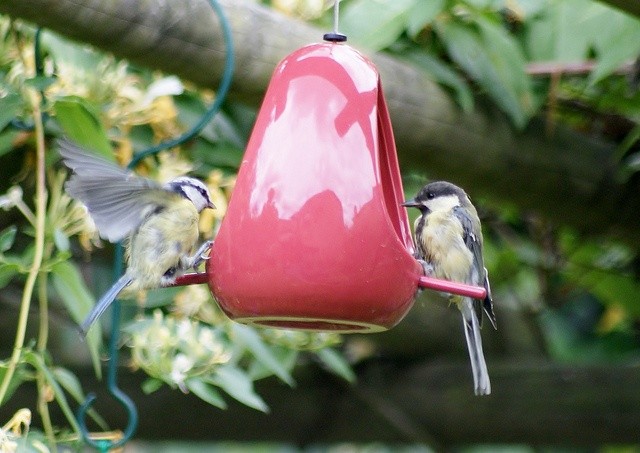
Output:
[401,180,497,396]
[55,135,217,337]
